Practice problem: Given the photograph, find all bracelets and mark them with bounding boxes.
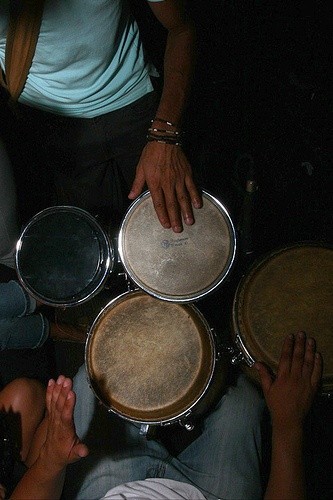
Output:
[144,115,184,148]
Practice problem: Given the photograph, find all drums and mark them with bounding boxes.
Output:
[14,205,120,310]
[123,191,237,307]
[231,243,333,400]
[84,285,216,426]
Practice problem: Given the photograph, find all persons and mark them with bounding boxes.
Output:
[13,326,322,500]
[0,279,94,500]
[0,0,205,234]
